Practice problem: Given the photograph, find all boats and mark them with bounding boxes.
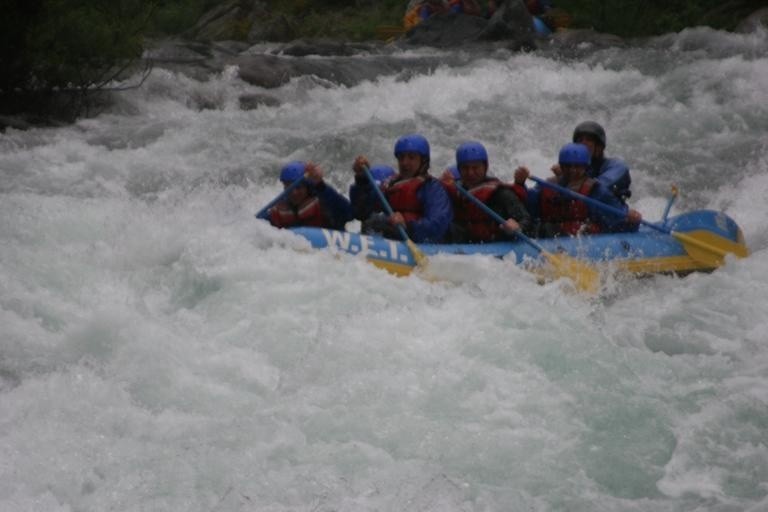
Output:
[285,209,748,284]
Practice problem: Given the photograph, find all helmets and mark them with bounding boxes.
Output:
[454,142,489,172]
[393,134,431,158]
[446,166,460,180]
[571,121,606,150]
[559,143,592,169]
[368,165,394,194]
[280,160,308,187]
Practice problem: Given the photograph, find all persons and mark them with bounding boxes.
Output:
[446,139,532,244]
[268,156,353,230]
[551,118,633,204]
[514,142,643,243]
[348,131,452,247]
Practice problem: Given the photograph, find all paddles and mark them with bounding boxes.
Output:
[528,174,750,269]
[453,182,601,294]
